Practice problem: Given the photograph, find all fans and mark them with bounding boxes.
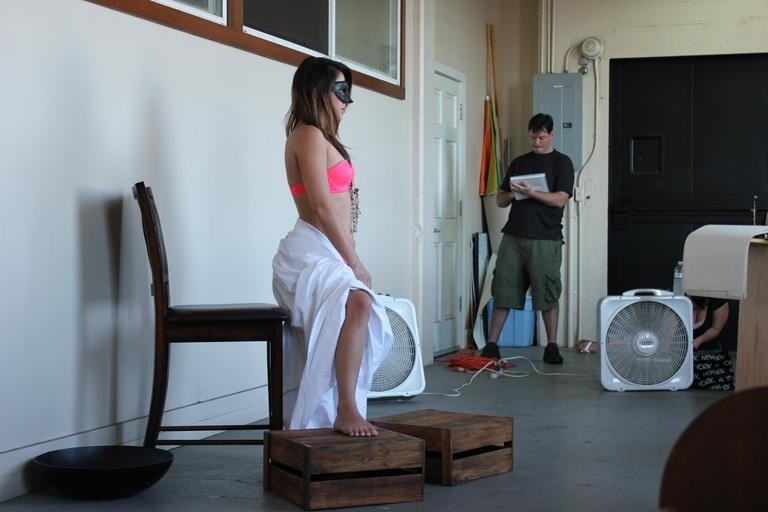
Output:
[365,294,425,400]
[596,287,694,393]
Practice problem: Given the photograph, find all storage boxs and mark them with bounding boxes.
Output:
[486,295,535,348]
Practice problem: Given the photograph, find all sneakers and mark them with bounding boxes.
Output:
[543,344,563,364]
[480,342,501,360]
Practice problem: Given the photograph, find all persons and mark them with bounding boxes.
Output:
[271,56,395,439]
[480,113,574,365]
[681,293,735,392]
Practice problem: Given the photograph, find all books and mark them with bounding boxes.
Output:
[509,172,550,202]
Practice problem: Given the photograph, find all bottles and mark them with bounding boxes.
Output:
[672,261,685,297]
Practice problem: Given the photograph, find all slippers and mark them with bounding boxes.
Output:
[578,340,596,353]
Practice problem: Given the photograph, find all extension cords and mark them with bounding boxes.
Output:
[496,367,503,375]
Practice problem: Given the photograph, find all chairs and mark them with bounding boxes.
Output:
[131,181,291,450]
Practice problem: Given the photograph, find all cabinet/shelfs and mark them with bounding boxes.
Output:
[607,51,767,351]
